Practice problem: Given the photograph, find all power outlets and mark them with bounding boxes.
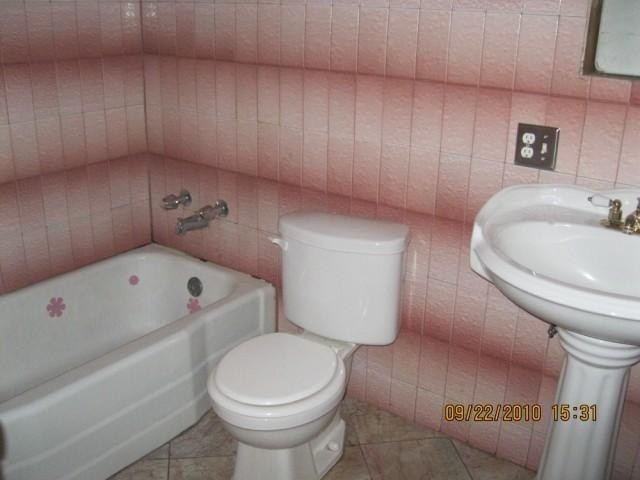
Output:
[514,122,560,171]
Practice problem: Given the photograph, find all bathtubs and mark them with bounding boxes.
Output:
[0,242,277,480]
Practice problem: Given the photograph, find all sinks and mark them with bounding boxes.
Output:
[468,182,640,346]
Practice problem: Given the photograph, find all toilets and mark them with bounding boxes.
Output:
[206,209,413,480]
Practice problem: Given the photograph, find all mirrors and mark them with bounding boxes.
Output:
[582,0,640,81]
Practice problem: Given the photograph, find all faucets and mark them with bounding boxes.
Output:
[622,197,640,232]
[174,213,209,237]
